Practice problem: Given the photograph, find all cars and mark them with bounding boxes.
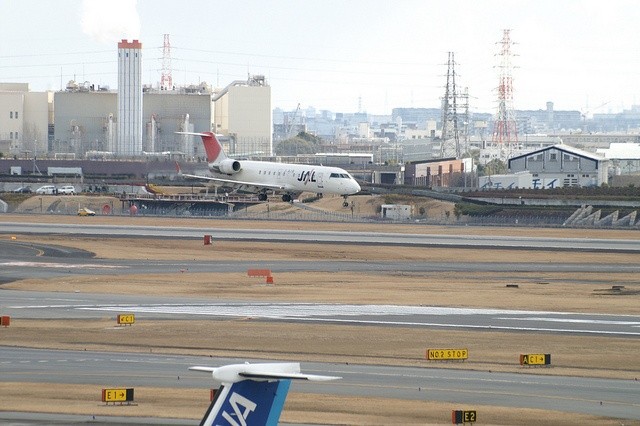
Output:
[14,186,31,193]
[77,208,95,216]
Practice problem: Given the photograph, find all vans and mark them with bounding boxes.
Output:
[57,185,75,195]
[35,185,56,195]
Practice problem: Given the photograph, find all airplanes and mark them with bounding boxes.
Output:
[187,360,343,425]
[174,130,361,208]
